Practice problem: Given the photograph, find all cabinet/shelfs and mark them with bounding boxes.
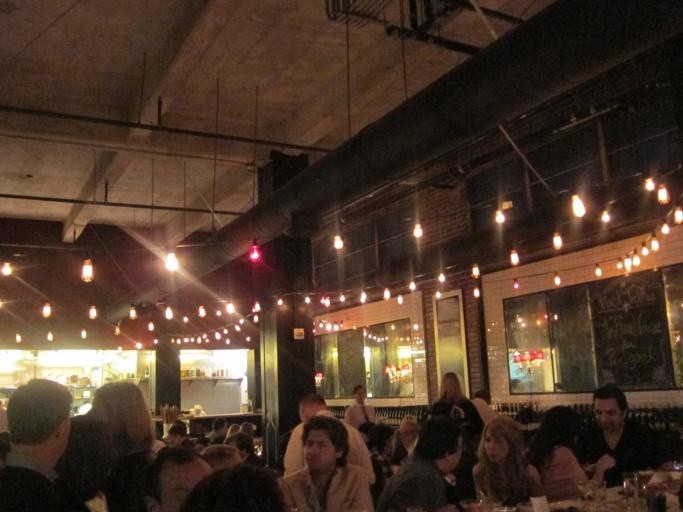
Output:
[0,384,98,401]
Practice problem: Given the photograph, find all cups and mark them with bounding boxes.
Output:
[621,470,650,511]
[584,480,607,512]
[240,404,248,413]
[457,498,516,512]
[516,501,533,511]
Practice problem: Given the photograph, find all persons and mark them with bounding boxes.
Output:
[278,393,377,511]
[344,385,375,428]
[358,416,462,512]
[0,378,92,511]
[151,418,288,511]
[471,384,683,505]
[53,377,152,511]
[433,371,483,502]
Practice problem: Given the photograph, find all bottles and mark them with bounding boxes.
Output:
[492,401,683,432]
[125,372,135,383]
[246,400,253,413]
[143,365,150,379]
[327,405,427,426]
[180,367,228,377]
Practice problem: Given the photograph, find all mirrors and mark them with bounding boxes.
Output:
[660,262,683,389]
[502,277,596,393]
[314,317,414,399]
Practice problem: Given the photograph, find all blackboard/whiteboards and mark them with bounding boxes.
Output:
[587,266,676,394]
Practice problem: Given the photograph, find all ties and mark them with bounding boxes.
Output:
[361,405,370,422]
